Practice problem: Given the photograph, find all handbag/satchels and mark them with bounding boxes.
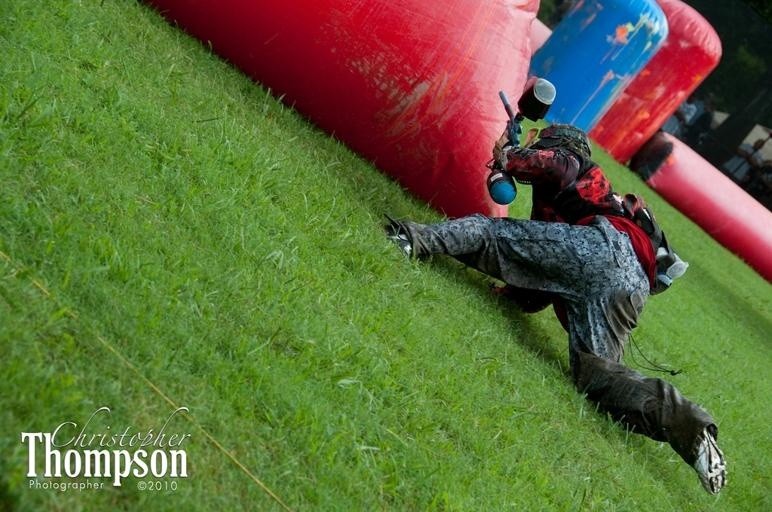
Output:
[605,190,689,297]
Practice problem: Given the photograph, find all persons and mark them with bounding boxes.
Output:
[687,88,709,128]
[724,136,765,182]
[379,119,730,496]
[657,92,698,137]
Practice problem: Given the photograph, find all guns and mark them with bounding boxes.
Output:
[488,78,556,205]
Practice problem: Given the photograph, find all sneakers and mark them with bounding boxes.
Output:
[384,223,412,259]
[688,426,727,495]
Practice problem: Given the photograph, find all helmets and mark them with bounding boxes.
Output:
[530,125,591,161]
